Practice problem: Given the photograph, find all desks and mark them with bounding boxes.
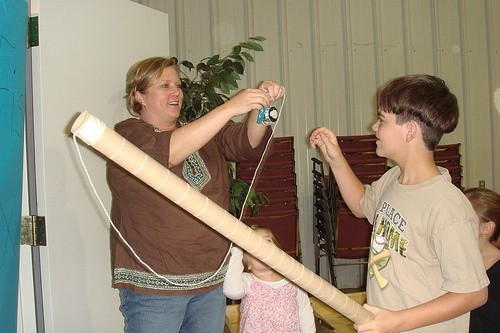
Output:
[223,292,367,333]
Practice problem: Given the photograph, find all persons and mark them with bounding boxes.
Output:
[308,75,491,333]
[105,57,286,333]
[221,224,317,333]
[462,189,500,333]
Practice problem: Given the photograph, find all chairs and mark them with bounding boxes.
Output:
[310,135,465,289]
[235,136,303,265]
